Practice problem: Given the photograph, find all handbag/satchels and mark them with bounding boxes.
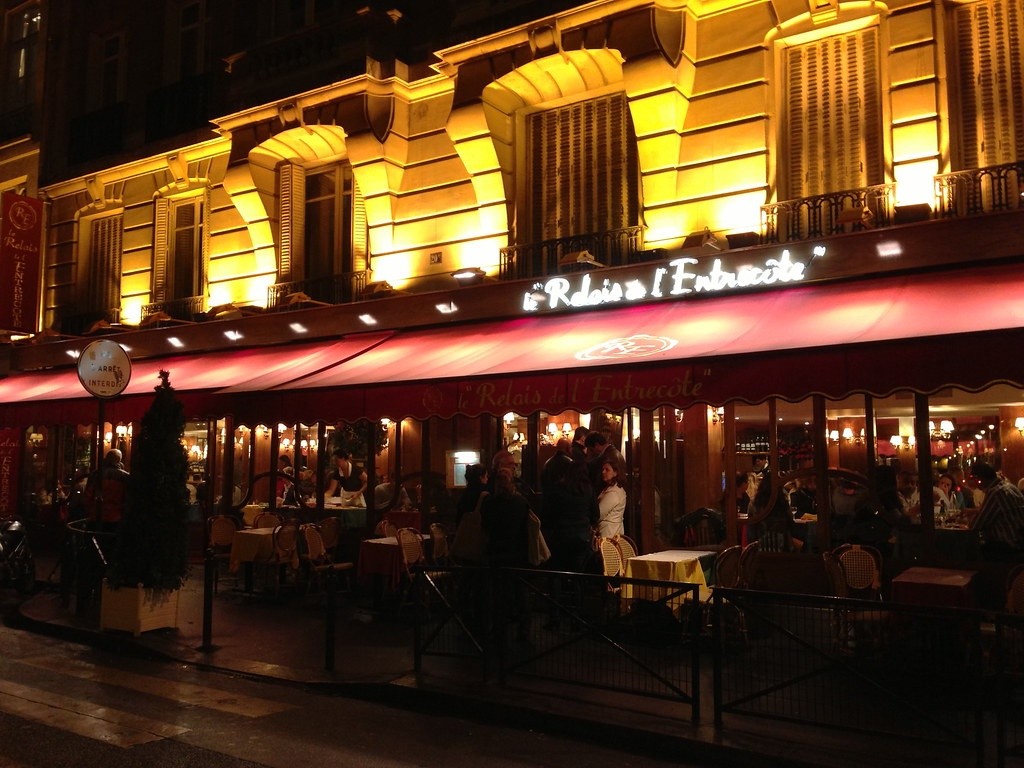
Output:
[528,509,551,565]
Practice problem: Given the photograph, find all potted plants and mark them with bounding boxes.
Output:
[99,370,194,638]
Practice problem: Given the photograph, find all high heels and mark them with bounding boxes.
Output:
[543,615,561,629]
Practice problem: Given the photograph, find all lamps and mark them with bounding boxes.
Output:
[80,320,134,336]
[361,281,412,296]
[33,329,82,341]
[278,292,334,307]
[206,305,258,316]
[558,251,606,269]
[838,208,875,232]
[682,231,724,253]
[140,313,197,326]
[451,268,497,283]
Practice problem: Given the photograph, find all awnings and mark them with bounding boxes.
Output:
[0,257,1024,426]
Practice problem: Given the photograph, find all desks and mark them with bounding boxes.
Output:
[902,518,974,567]
[230,526,303,596]
[621,550,711,612]
[737,512,749,542]
[356,534,431,601]
[892,567,975,628]
[793,519,819,553]
[243,505,264,526]
[306,501,367,531]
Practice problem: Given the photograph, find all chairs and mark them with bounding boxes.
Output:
[823,544,894,648]
[679,541,760,647]
[598,533,638,619]
[205,512,354,601]
[396,522,457,601]
[987,563,1024,669]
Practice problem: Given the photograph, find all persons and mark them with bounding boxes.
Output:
[32,425,1024,664]
